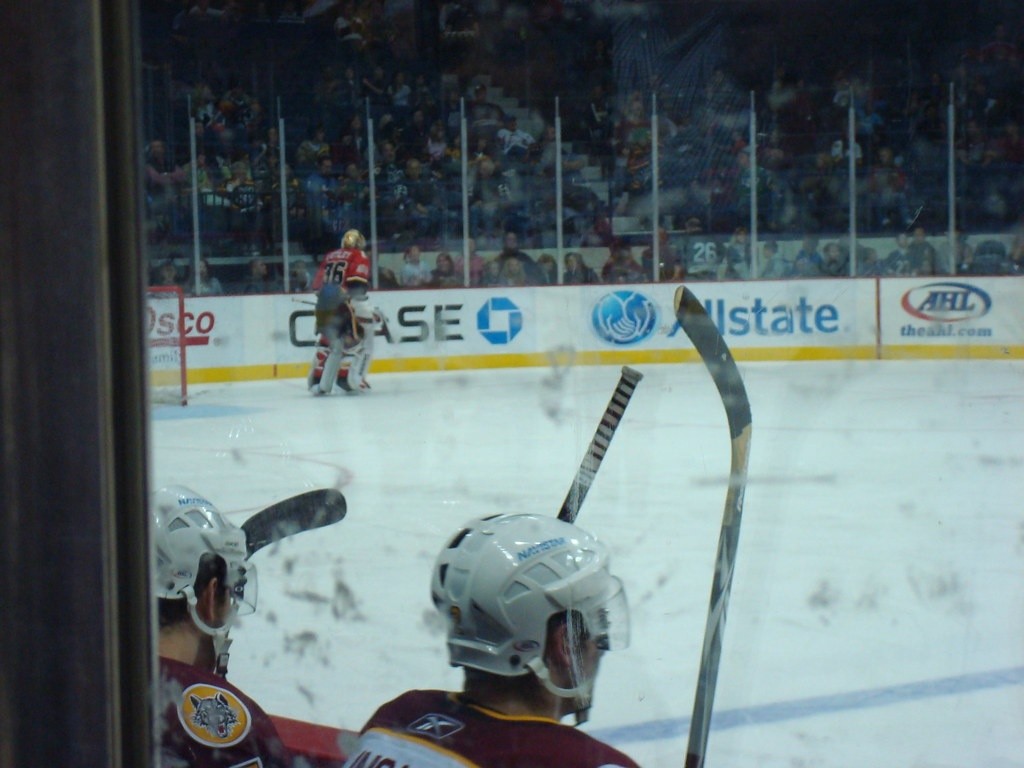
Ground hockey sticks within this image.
[672,282,753,768]
[290,295,318,306]
[552,362,644,526]
[243,487,348,562]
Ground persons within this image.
[151,485,296,768]
[343,513,645,768]
[309,229,371,393]
[137,1,1024,292]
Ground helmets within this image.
[341,229,366,250]
[431,511,620,676]
[151,486,248,599]
